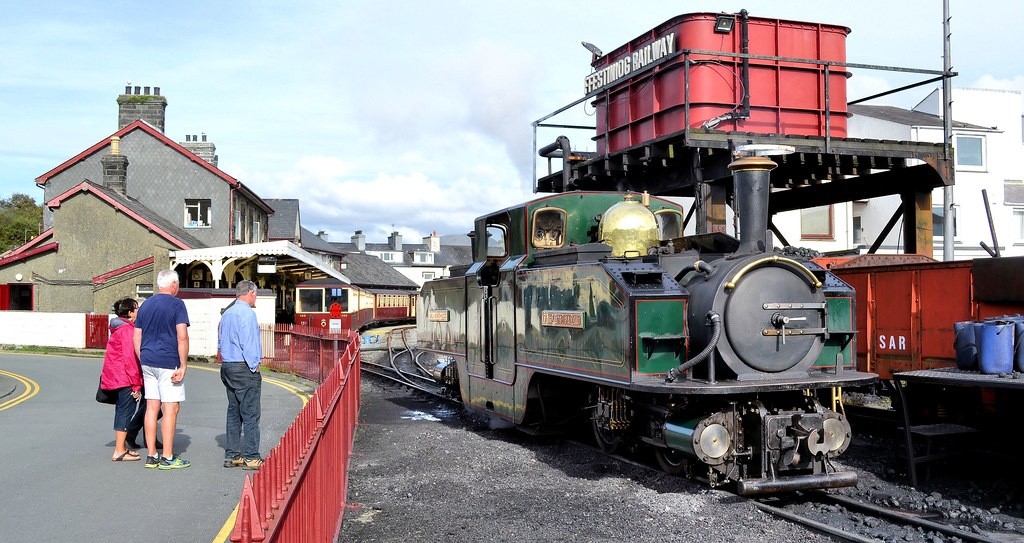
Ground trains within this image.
[295,278,421,331]
[416,158,877,495]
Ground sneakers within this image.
[144,455,160,468]
[223,456,244,467]
[242,458,263,470]
[158,453,190,470]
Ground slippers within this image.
[112,449,141,461]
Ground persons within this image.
[133,267,190,469]
[217,279,266,470]
[100,297,164,462]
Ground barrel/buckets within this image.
[953,314,1024,375]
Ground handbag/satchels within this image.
[96,375,119,404]
